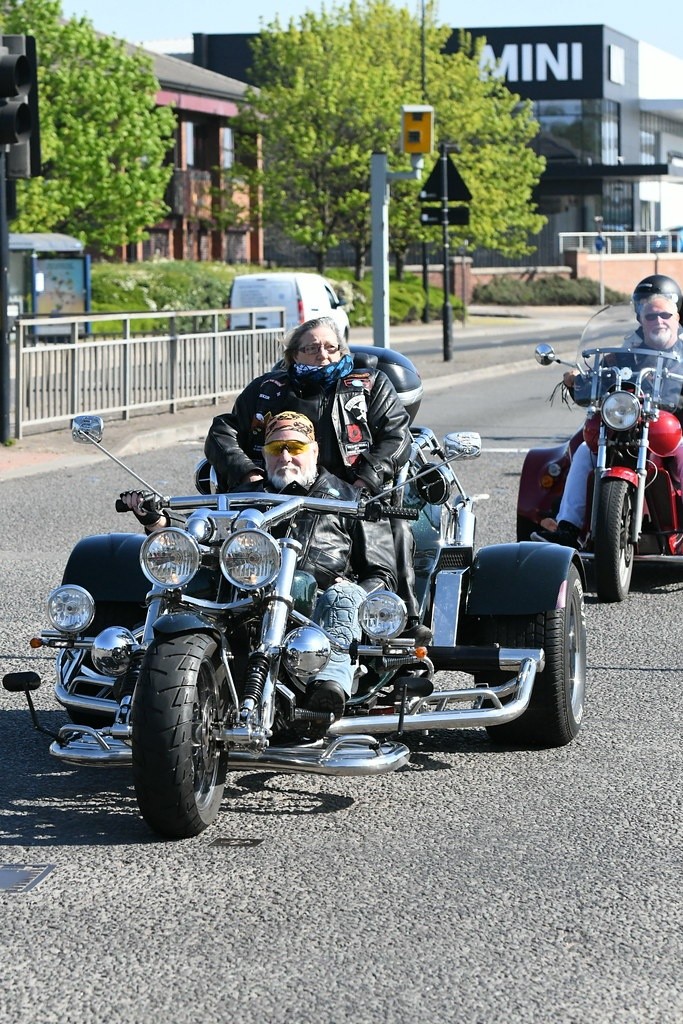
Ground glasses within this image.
[295,344,340,354]
[263,441,310,455]
[643,312,673,320]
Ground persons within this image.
[603,274,682,348]
[530,294,683,559]
[119,412,396,741]
[204,316,433,649]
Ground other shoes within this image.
[308,681,346,738]
[396,625,433,647]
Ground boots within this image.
[530,520,586,552]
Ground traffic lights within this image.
[0,45,31,148]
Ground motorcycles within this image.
[514,302,682,605]
[0,337,590,842]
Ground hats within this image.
[263,410,315,444]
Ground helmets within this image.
[646,410,681,457]
[631,275,682,314]
[583,411,614,455]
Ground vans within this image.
[227,271,351,362]
